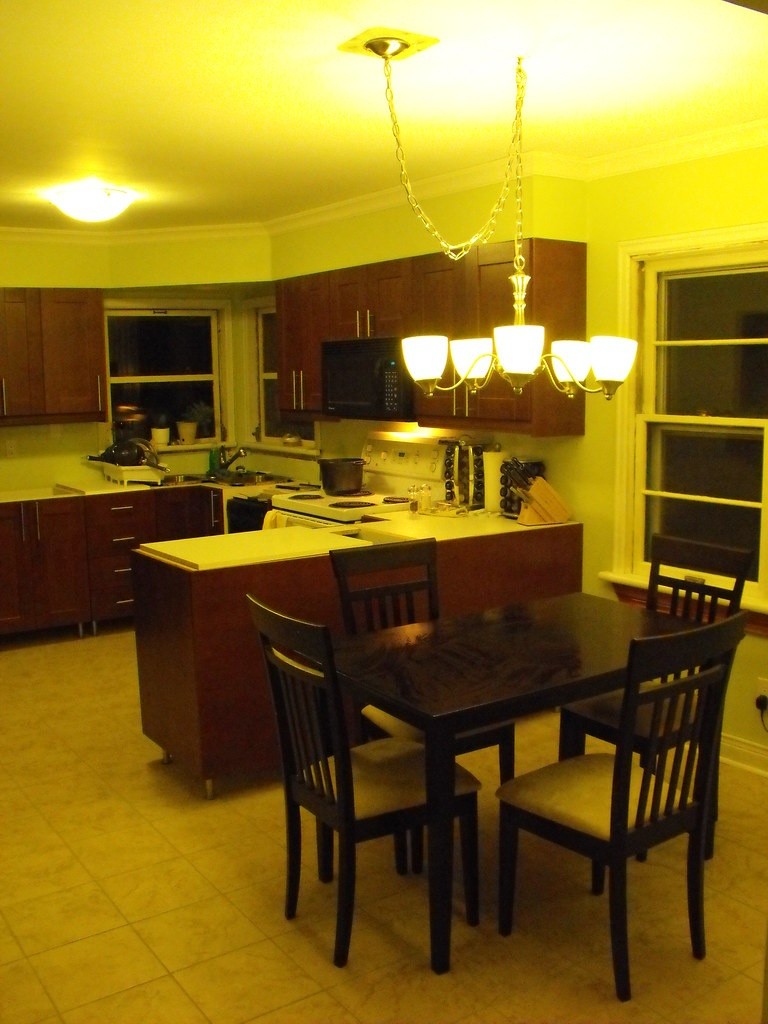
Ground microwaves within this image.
[320,337,416,422]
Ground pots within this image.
[85,438,170,473]
[317,457,366,496]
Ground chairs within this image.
[496,611,749,1001]
[560,536,751,858]
[244,537,517,968]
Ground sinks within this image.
[162,475,201,483]
[219,472,292,485]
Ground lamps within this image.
[337,26,638,402]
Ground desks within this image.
[295,593,708,976]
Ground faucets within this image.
[219,445,248,474]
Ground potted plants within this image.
[150,413,170,446]
[176,402,213,444]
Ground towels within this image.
[262,510,289,530]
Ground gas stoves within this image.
[271,428,457,528]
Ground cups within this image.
[176,421,197,445]
[151,427,170,447]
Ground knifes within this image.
[501,456,536,503]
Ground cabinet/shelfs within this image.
[412,237,586,438]
[0,286,109,427]
[156,486,224,542]
[331,257,412,341]
[275,271,339,422]
[84,489,156,637]
[129,524,584,801]
[0,496,92,639]
